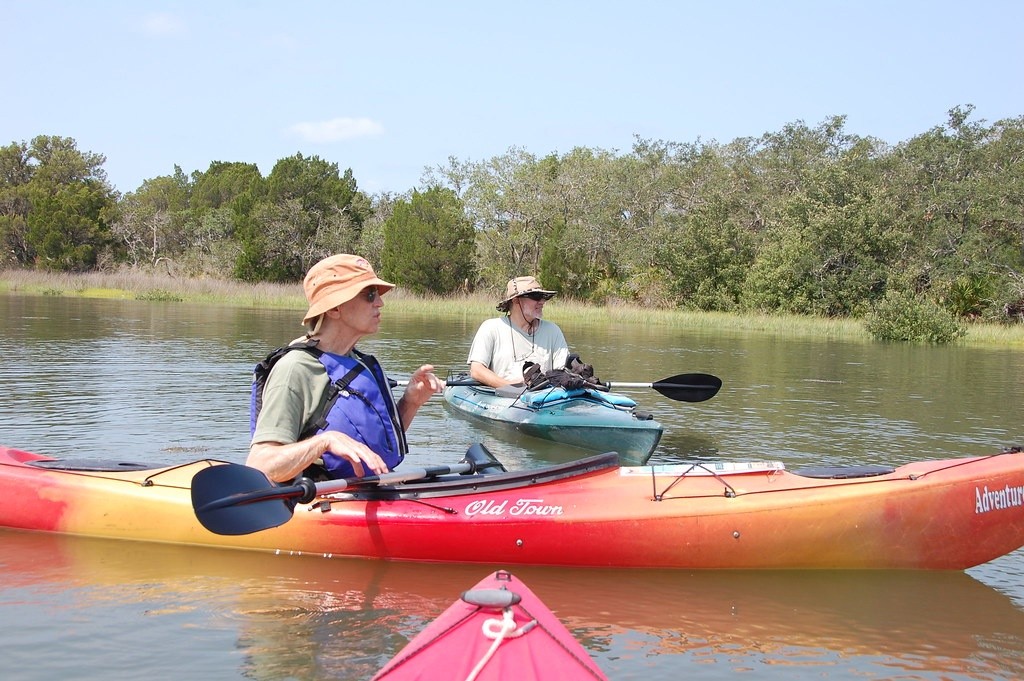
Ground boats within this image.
[369,570,608,681]
[440,373,665,466]
[0,438,1024,574]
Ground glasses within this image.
[518,292,553,302]
[360,286,380,303]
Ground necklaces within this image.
[507,315,535,362]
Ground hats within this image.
[495,275,558,313]
[300,254,397,327]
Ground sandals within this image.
[563,353,612,392]
[522,361,551,392]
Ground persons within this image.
[245,253,446,483]
[465,276,570,392]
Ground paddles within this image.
[189,442,509,538]
[386,372,724,403]
